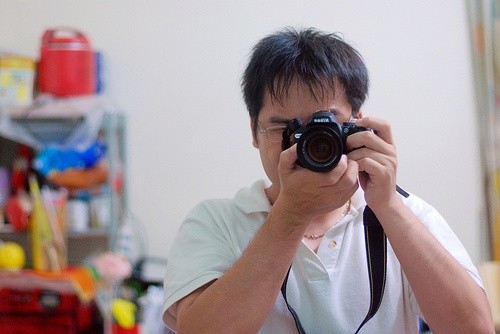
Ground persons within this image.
[161,27,496,334]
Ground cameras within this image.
[282,110,374,173]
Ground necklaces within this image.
[267,183,351,240]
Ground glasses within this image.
[257,120,288,142]
